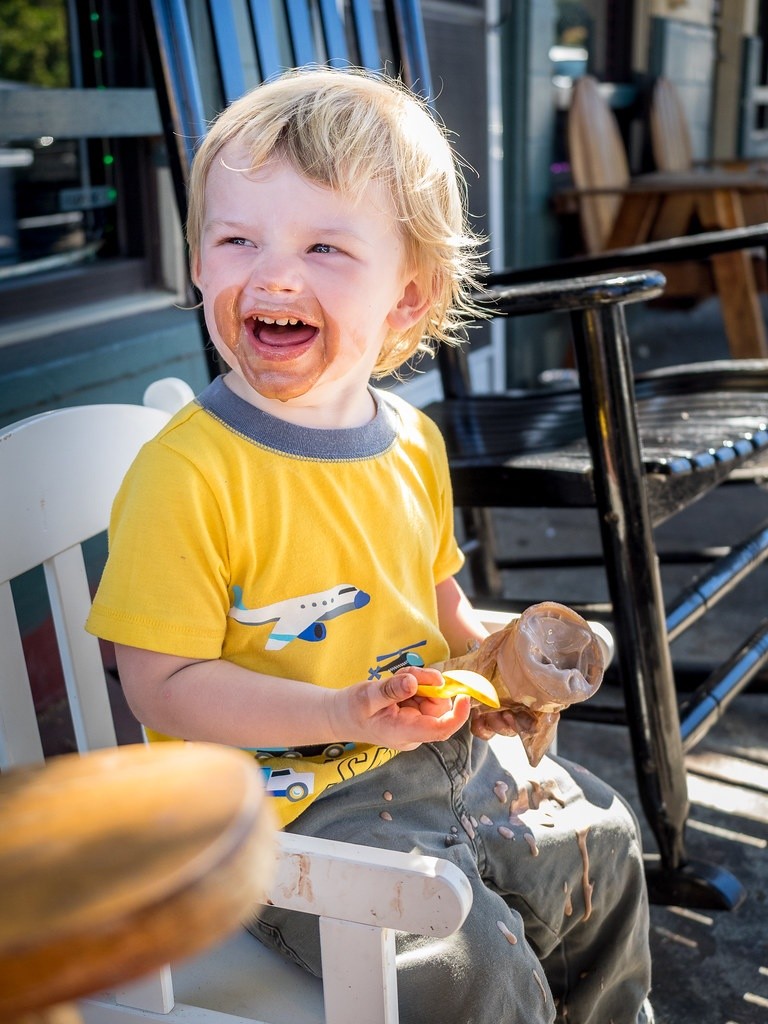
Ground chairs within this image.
[0,378,614,1023]
[140,1,768,908]
[647,73,767,315]
[569,71,768,362]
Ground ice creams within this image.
[424,602,602,766]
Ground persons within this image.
[82,57,659,1024]
